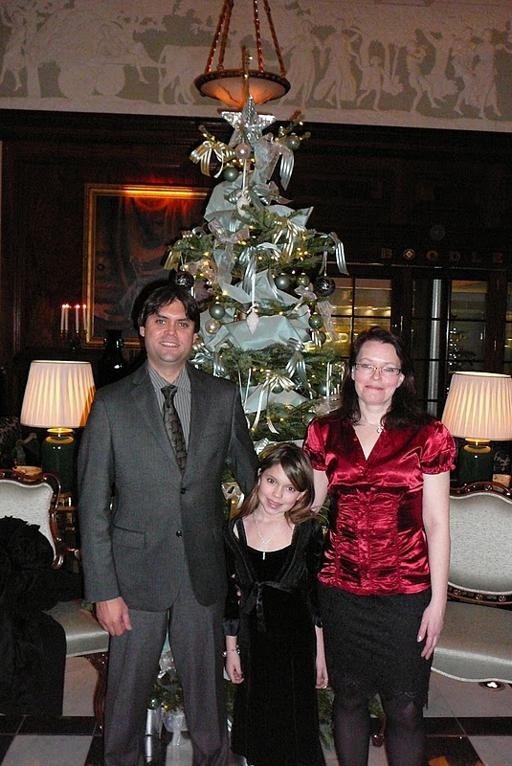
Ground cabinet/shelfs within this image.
[303,251,511,421]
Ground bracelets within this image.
[226,648,237,652]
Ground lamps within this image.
[18,359,96,491]
[436,369,512,494]
[192,1,294,112]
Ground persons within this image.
[226,439,331,763]
[298,328,458,765]
[73,277,263,764]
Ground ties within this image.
[161,386,186,478]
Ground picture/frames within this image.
[74,180,224,355]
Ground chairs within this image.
[368,492,512,749]
[0,468,112,734]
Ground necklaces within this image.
[362,413,384,434]
[250,509,284,561]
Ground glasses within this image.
[353,364,401,375]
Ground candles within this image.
[59,299,89,334]
